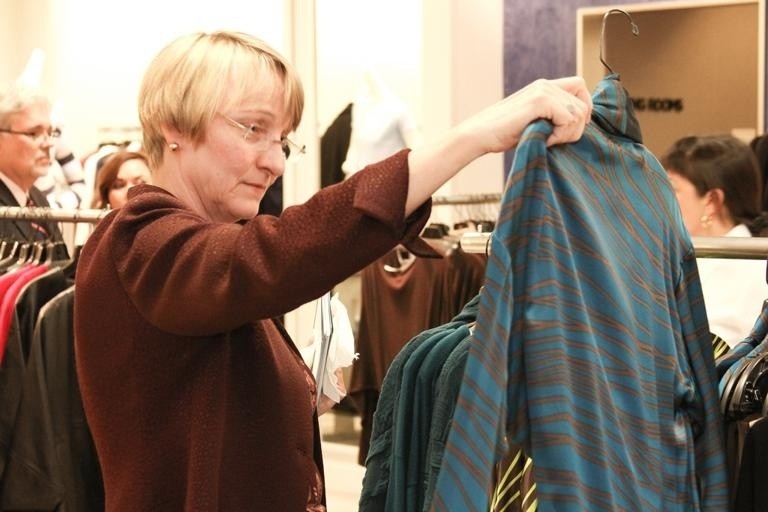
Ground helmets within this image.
[215,109,307,162]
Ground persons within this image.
[659,134,768,351]
[0,87,157,261]
[72,30,591,512]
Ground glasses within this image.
[0,127,61,142]
